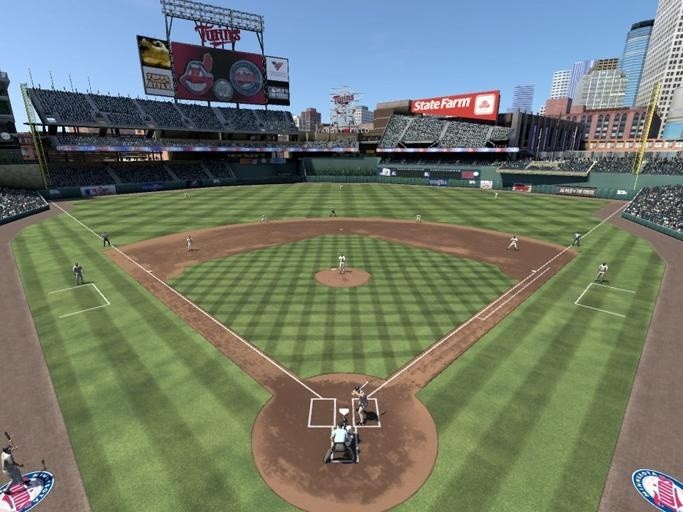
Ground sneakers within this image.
[24,480,30,484]
[4,491,11,495]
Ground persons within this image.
[622,184,683,239]
[0,183,46,223]
[351,385,368,425]
[73,262,84,284]
[308,135,377,176]
[571,232,582,247]
[337,252,346,273]
[333,416,355,450]
[515,148,683,175]
[506,234,520,250]
[25,82,305,189]
[261,214,266,221]
[595,263,609,280]
[101,231,111,247]
[323,420,357,463]
[416,213,422,223]
[330,208,336,217]
[1,445,31,494]
[495,191,499,199]
[378,110,515,176]
[187,234,193,252]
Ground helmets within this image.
[355,386,360,390]
[3,445,11,453]
[343,417,347,423]
